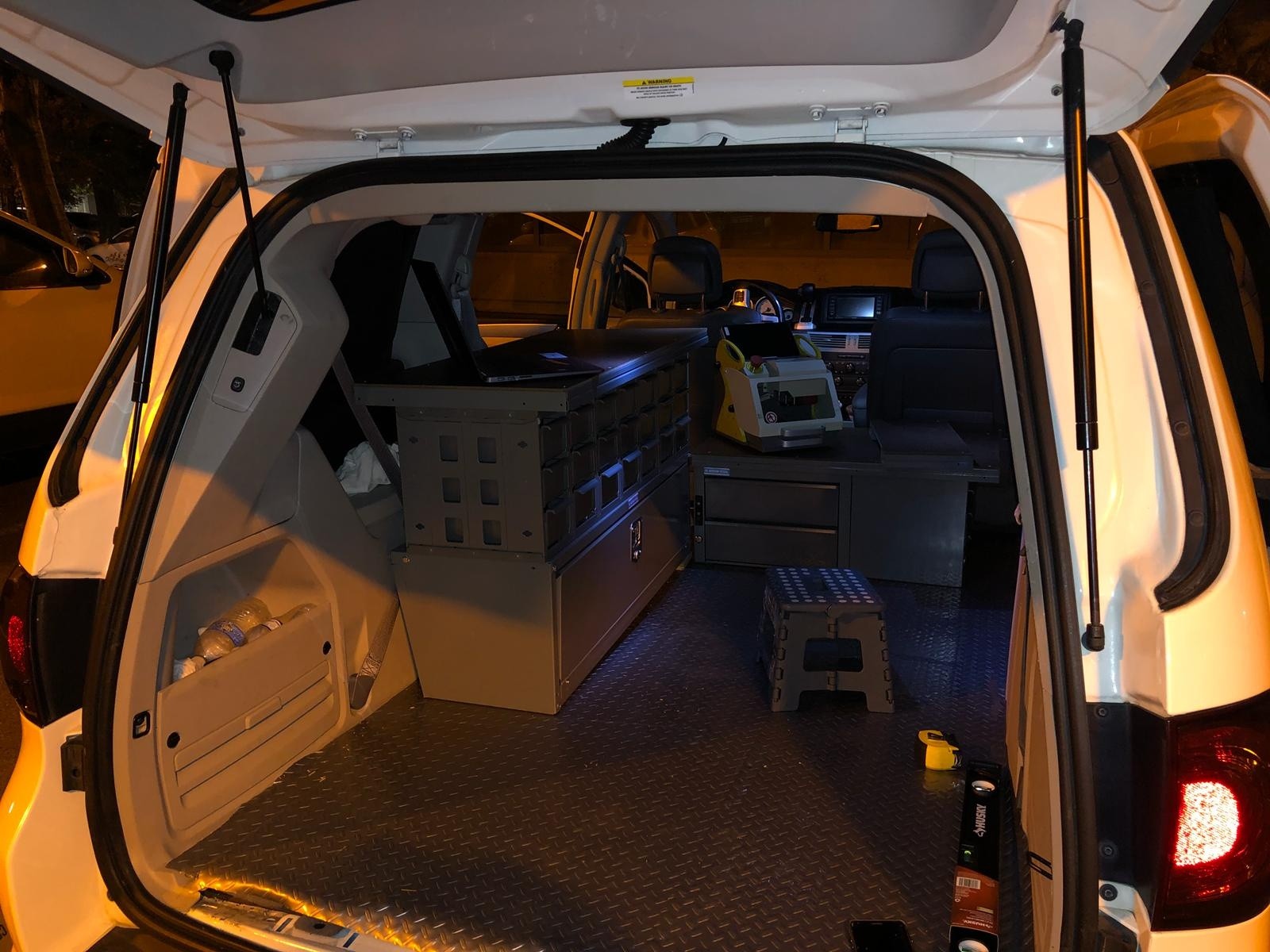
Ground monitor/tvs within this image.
[827,295,887,321]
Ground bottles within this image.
[190,597,271,662]
[232,603,317,651]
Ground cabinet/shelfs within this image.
[351,328,710,714]
[693,421,998,588]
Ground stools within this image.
[757,566,895,713]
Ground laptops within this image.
[410,261,605,386]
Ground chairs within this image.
[616,235,760,341]
[869,230,1007,418]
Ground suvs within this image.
[0,0,1269,950]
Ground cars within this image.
[622,211,721,275]
[0,207,125,486]
[85,226,135,272]
[68,220,100,251]
